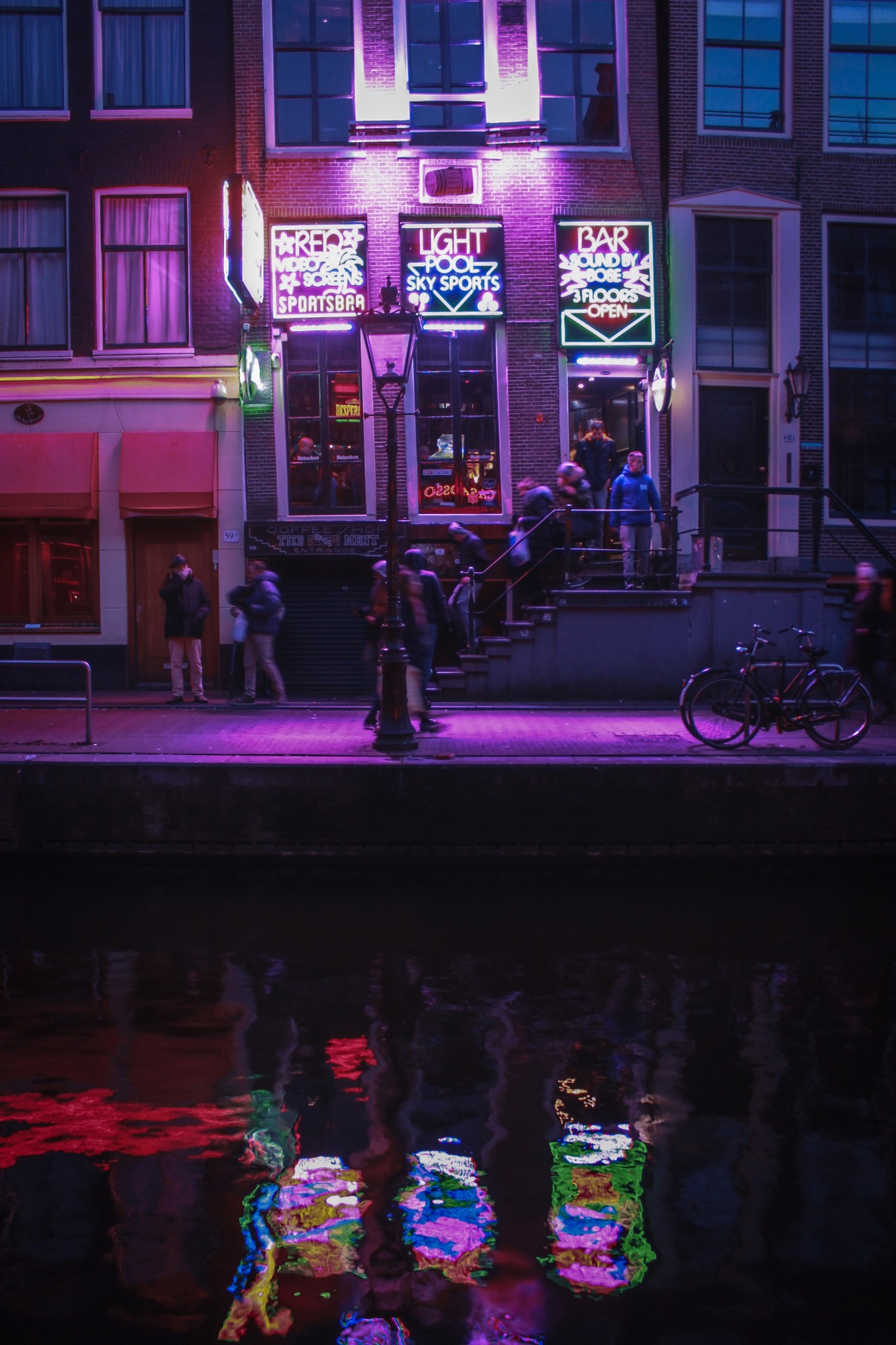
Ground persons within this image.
[609,451,665,590]
[227,561,286,704]
[420,445,430,460]
[837,562,887,723]
[289,437,343,516]
[573,420,620,552]
[365,549,446,731]
[512,477,556,607]
[556,461,599,590]
[230,579,285,703]
[448,521,488,654]
[159,554,211,704]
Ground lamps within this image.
[783,355,813,423]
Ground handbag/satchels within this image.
[232,610,248,643]
[509,522,530,566]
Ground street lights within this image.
[349,273,423,752]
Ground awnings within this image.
[0,432,99,519]
[119,431,219,519]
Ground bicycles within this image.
[677,623,876,751]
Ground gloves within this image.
[179,569,189,580]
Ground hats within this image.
[169,554,186,568]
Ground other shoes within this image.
[194,695,209,703]
[363,718,377,729]
[233,694,255,704]
[625,580,634,589]
[165,696,183,704]
[419,719,441,731]
[271,695,288,704]
[635,579,644,588]
